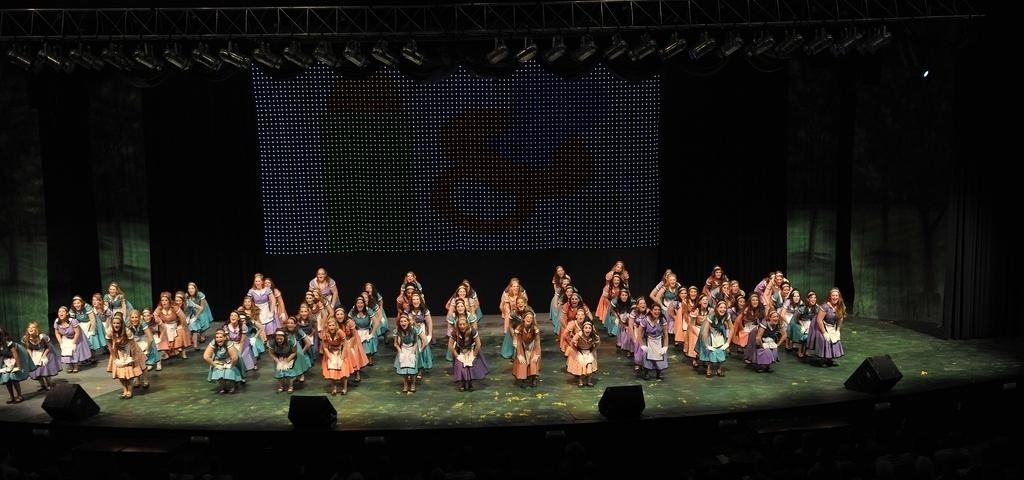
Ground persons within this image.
[2,260,847,405]
[331,418,1008,480]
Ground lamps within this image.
[598,385,646,424]
[39,379,101,425]
[285,391,340,430]
[844,350,904,402]
[4,0,898,79]
